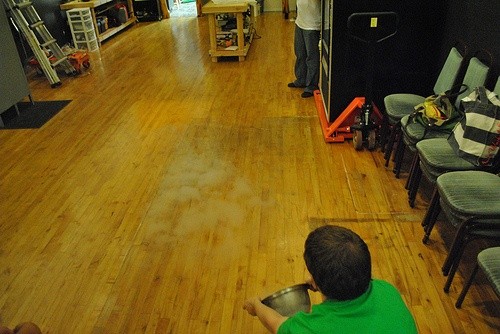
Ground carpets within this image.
[0,100,73,130]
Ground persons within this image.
[288,0,323,98]
[242,225,418,334]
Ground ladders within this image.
[1,0,77,89]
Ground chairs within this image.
[384,47,500,309]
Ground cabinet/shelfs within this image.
[66,7,99,52]
[201,0,257,62]
[60,0,137,48]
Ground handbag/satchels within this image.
[402,81,469,132]
[446,84,500,166]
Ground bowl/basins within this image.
[260,283,318,317]
